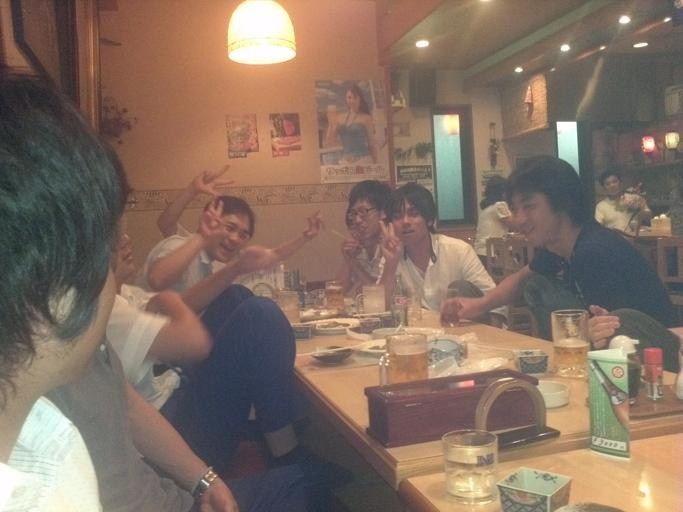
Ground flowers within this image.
[612,181,648,211]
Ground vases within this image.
[629,213,638,230]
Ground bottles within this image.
[606,334,639,407]
[641,344,664,400]
[588,359,632,436]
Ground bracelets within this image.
[193,468,219,498]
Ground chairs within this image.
[485,233,541,339]
[654,236,681,307]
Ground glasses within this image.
[348,207,379,220]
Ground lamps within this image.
[225,0,298,69]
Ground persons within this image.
[0,71,128,512]
[96,209,356,490]
[47,336,238,511]
[323,81,379,165]
[594,172,652,233]
[132,162,328,436]
[328,175,519,328]
[440,157,679,374]
[273,118,300,156]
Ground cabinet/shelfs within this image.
[500,63,655,141]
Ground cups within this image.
[440,428,498,506]
[548,308,591,378]
[269,261,469,369]
[378,332,429,385]
[652,217,671,237]
[325,104,335,126]
